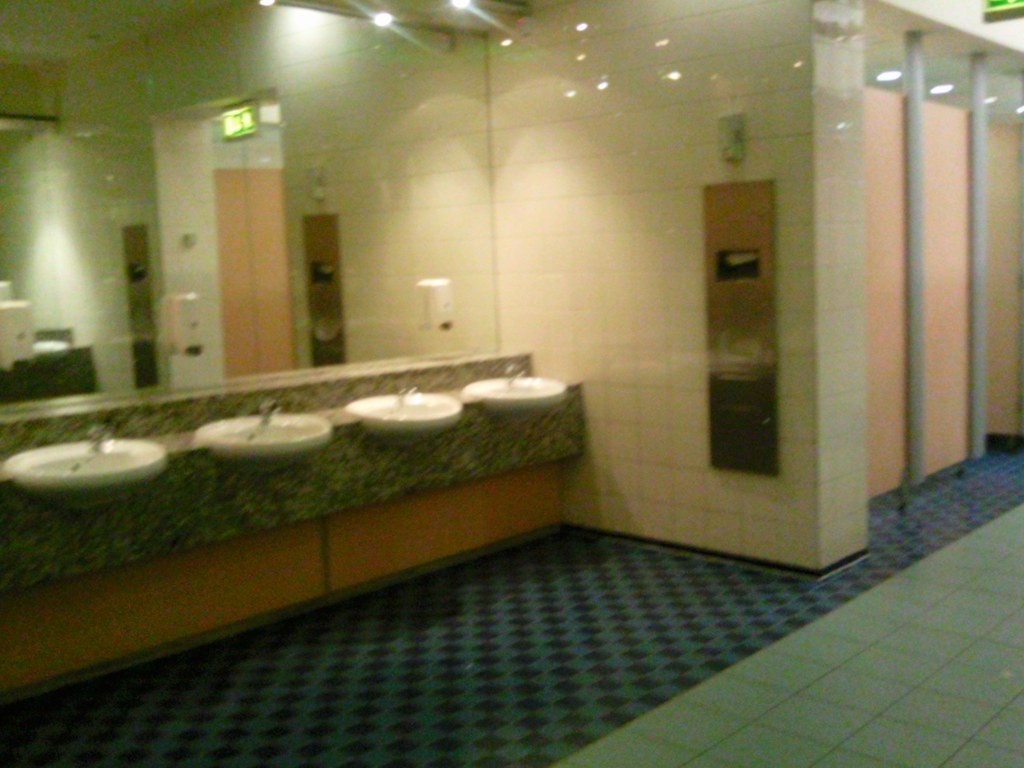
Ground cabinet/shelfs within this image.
[1,456,572,708]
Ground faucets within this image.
[85,421,118,458]
[395,384,420,409]
[259,397,283,429]
[506,368,527,390]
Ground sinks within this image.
[461,377,569,410]
[191,414,336,456]
[5,440,168,489]
[347,393,465,435]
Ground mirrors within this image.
[1,0,497,411]
